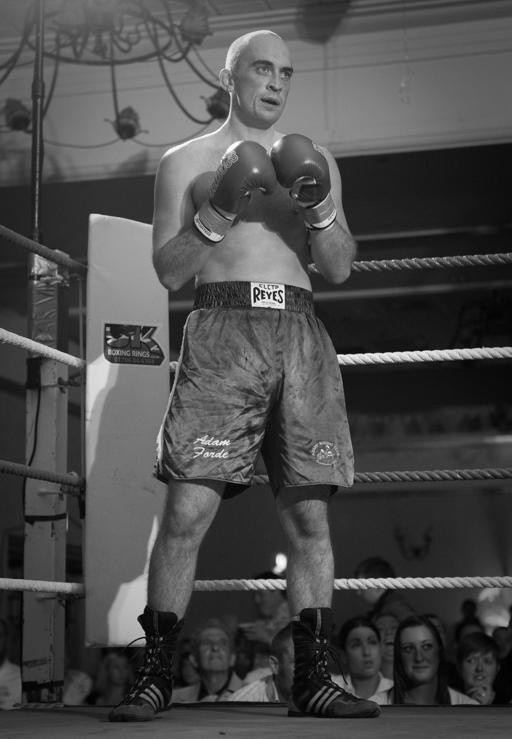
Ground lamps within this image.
[0,0,230,148]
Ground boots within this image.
[108,605,186,722]
[287,606,383,719]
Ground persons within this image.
[145,554,511,705]
[108,28,382,719]
[97,652,136,705]
